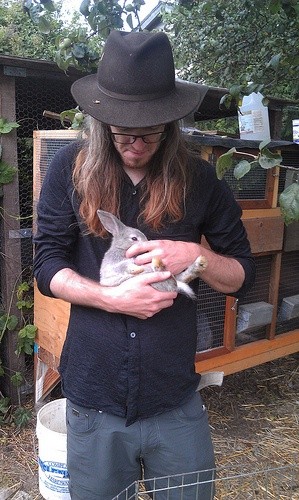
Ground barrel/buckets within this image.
[32,396,74,500]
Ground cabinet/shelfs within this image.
[35,129,299,407]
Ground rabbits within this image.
[97,209,208,300]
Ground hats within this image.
[70,30,209,129]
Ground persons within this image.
[33,30,255,500]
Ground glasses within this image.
[105,125,170,147]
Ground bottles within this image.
[238,81,272,143]
